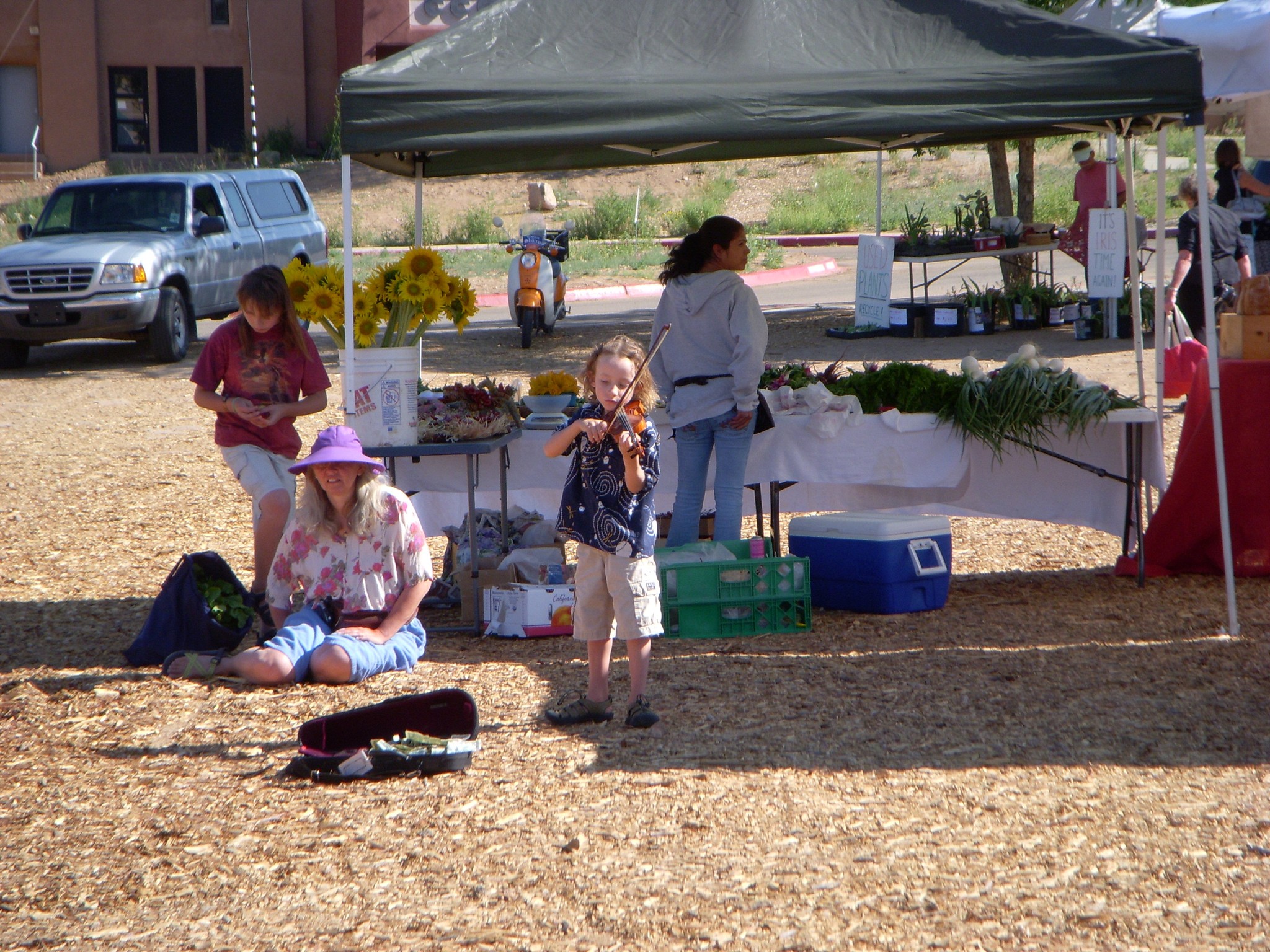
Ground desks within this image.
[874,244,1080,305]
[1115,358,1270,587]
[359,368,1161,584]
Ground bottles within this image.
[749,532,765,558]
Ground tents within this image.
[1156,0,1270,503]
[337,0,1242,637]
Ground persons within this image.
[162,188,207,229]
[190,263,332,632]
[162,424,434,688]
[1066,141,1126,322]
[1164,172,1252,344]
[1232,159,1270,275]
[1208,139,1257,278]
[543,335,665,728]
[647,214,769,547]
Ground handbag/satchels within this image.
[128,550,255,658]
[757,381,865,438]
[1227,168,1267,219]
[439,503,572,584]
[420,577,461,608]
[1163,305,1209,399]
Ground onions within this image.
[961,344,1109,392]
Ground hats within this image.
[288,426,388,474]
[1072,145,1093,162]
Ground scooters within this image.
[492,210,575,349]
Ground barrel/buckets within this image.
[925,303,965,337]
[888,303,924,338]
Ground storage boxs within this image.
[440,527,573,639]
[1219,312,1270,358]
[789,512,956,619]
[645,536,816,638]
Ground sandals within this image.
[547,695,614,722]
[624,694,659,726]
[164,649,231,680]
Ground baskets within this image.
[658,556,813,638]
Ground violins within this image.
[605,398,650,460]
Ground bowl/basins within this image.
[522,394,571,413]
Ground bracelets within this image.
[232,397,241,414]
[222,394,229,413]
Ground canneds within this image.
[750,535,764,558]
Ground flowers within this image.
[280,244,482,347]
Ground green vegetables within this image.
[193,563,256,630]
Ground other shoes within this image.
[257,628,276,647]
[249,588,275,625]
[1173,401,1187,412]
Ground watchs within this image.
[1166,284,1180,292]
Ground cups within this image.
[1058,227,1066,241]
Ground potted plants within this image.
[825,278,1165,340]
[892,191,1025,253]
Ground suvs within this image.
[0,168,330,371]
